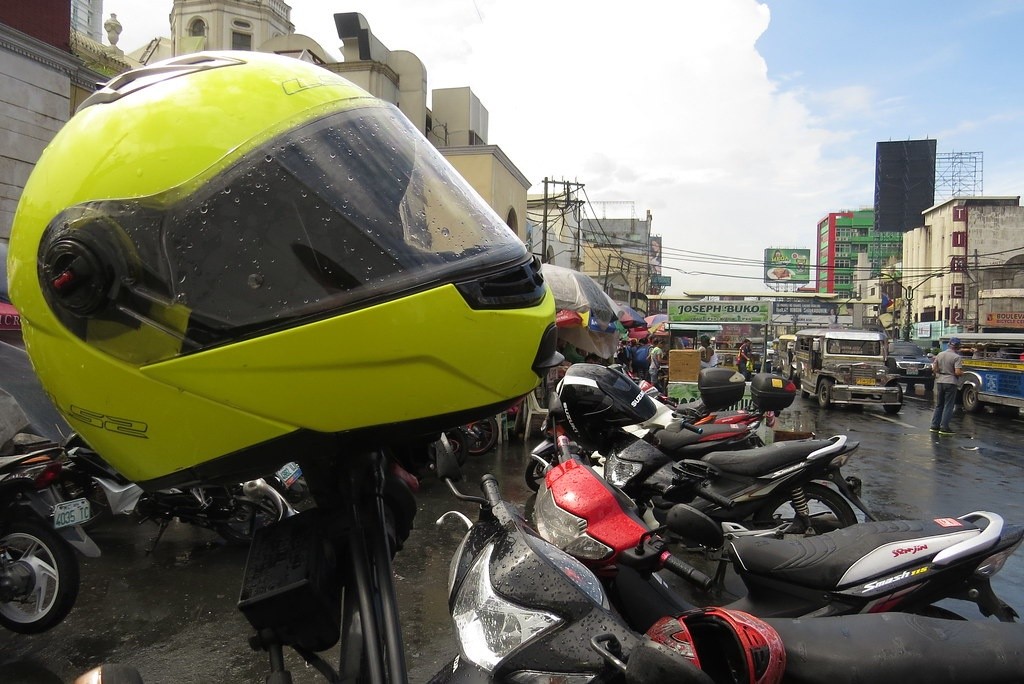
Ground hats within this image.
[950,337,960,347]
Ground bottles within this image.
[746,358,753,371]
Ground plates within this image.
[767,268,795,281]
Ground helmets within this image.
[7,50,565,491]
[644,606,786,684]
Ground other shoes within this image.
[939,427,956,435]
[929,428,940,433]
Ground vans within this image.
[794,329,904,415]
[938,334,1024,416]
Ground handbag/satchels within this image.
[746,360,754,372]
[659,369,665,375]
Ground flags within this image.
[881,291,893,311]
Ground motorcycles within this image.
[0,447,101,637]
[58,409,500,559]
[421,363,1024,684]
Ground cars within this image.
[887,342,936,391]
[744,334,799,380]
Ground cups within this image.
[796,259,806,272]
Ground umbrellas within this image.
[541,263,668,335]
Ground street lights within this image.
[814,294,862,324]
[877,273,948,344]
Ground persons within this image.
[616,335,714,386]
[932,337,964,435]
[737,338,752,381]
